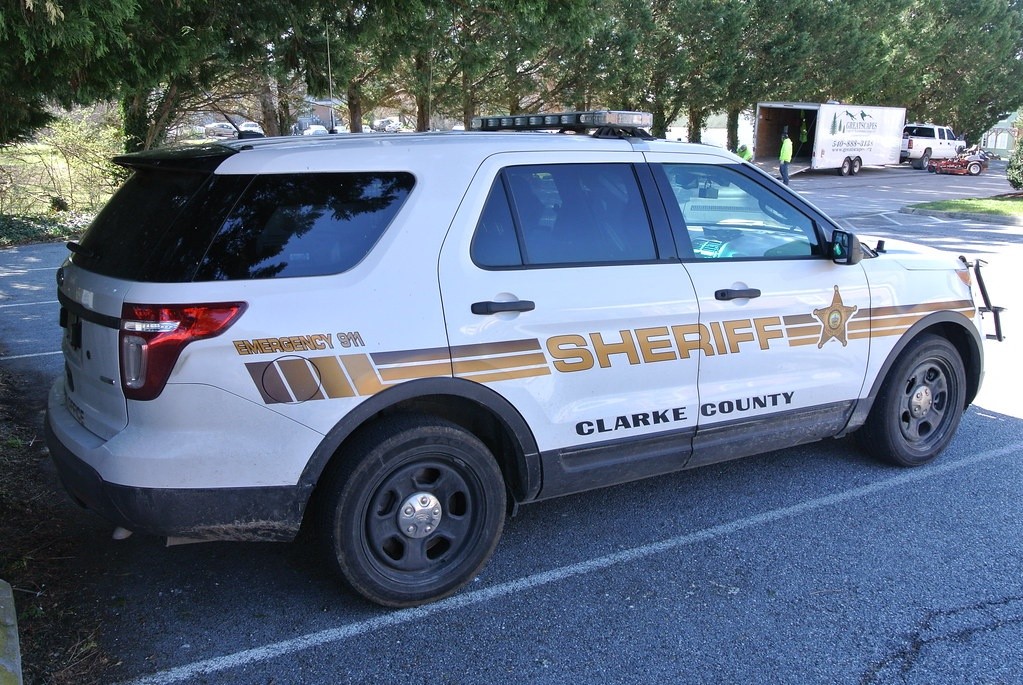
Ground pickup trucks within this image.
[899,122,967,170]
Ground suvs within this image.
[40,109,1007,610]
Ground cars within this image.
[984,151,1001,161]
[165,111,416,138]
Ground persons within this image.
[735,145,752,162]
[779,134,792,185]
